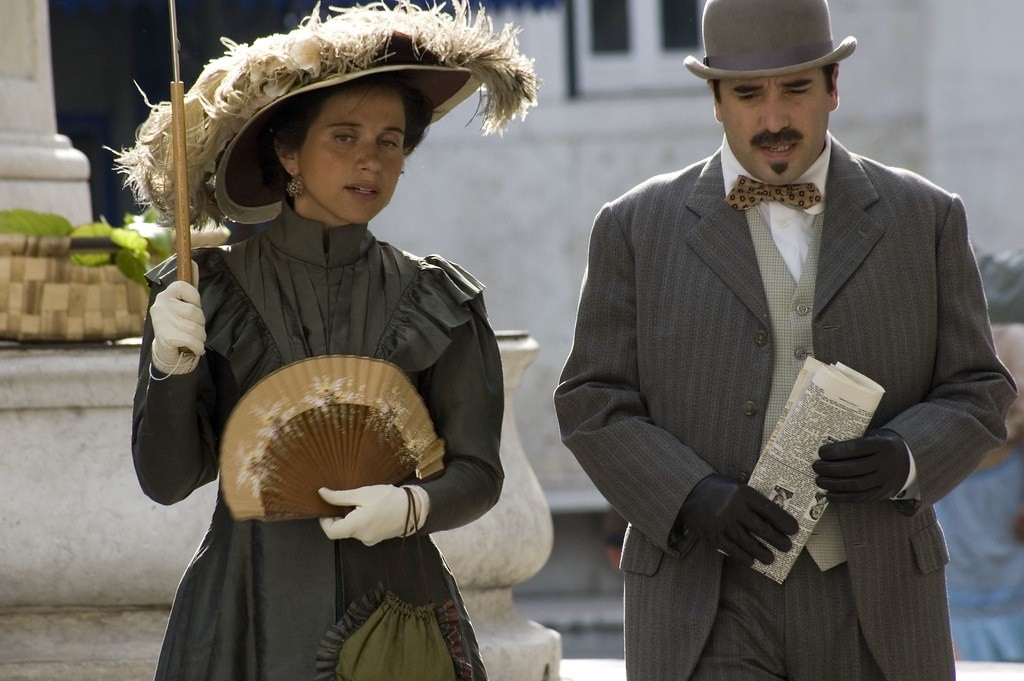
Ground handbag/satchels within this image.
[315,488,473,680]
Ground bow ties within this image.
[725,175,824,211]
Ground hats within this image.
[684,0,857,78]
[123,0,540,225]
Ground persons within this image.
[553,0,1018,680]
[115,1,537,680]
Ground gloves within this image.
[316,483,428,547]
[150,260,207,376]
[812,427,909,504]
[678,473,800,567]
